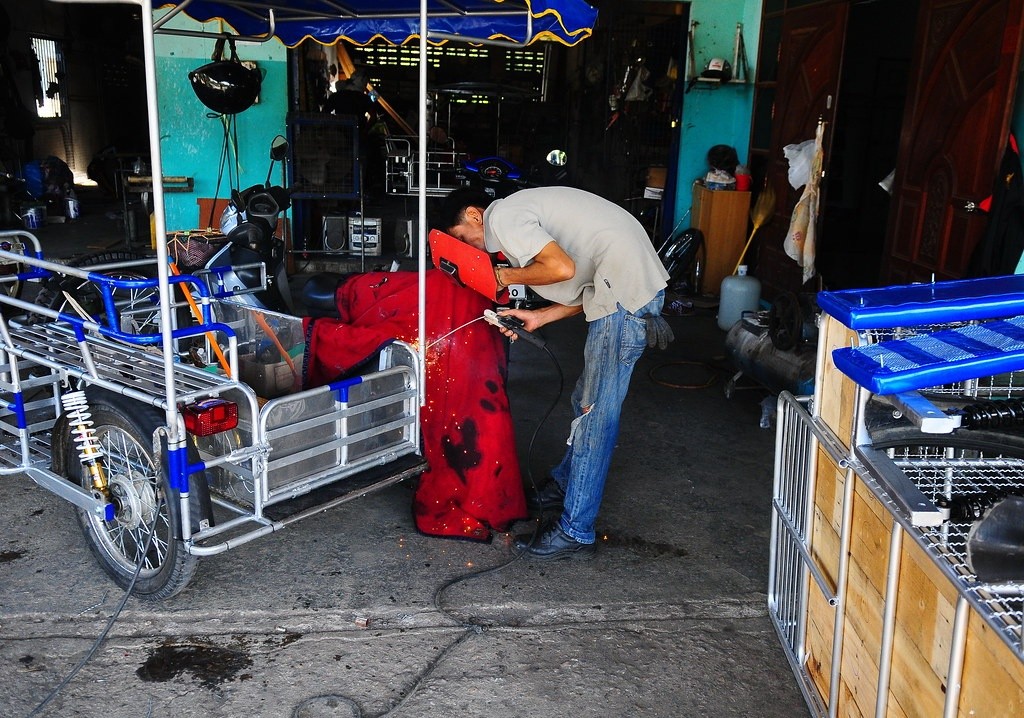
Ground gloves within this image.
[642,313,675,349]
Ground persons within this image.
[441,187,671,561]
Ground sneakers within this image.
[511,519,598,562]
[526,476,565,510]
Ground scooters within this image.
[176,135,363,352]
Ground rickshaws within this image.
[1,0,539,607]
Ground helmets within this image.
[188,60,262,114]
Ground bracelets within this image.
[496,267,507,287]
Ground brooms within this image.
[252,311,302,393]
[732,190,775,275]
[167,256,268,412]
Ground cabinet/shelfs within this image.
[690,184,751,297]
